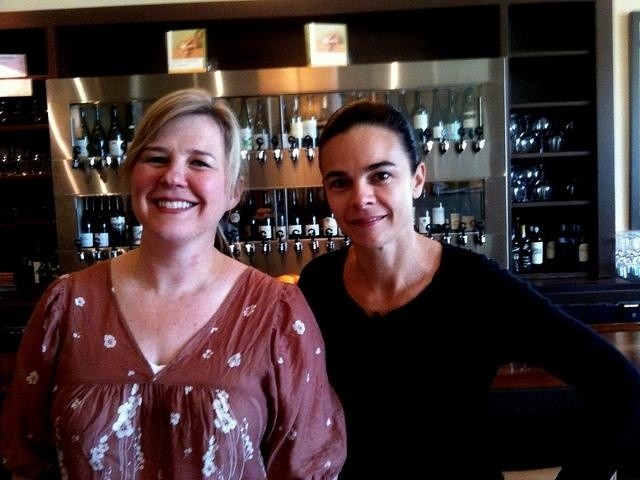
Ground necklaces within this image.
[371,309,382,317]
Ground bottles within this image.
[76,184,487,250]
[506,102,595,275]
[66,85,487,166]
[0,87,52,177]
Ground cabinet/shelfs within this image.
[0,0,617,301]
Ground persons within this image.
[6,91,350,480]
[296,94,639,480]
